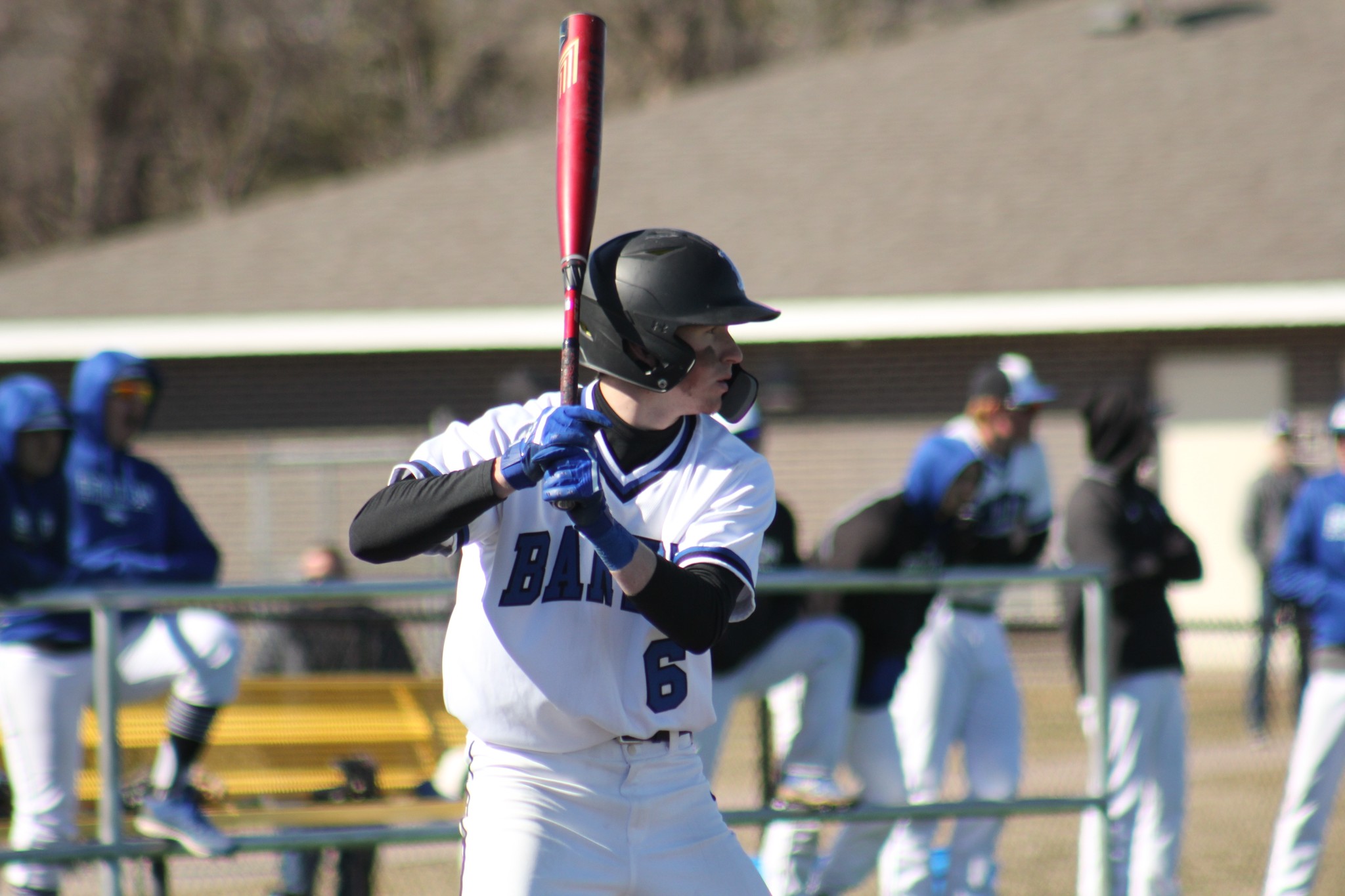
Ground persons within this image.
[0,352,240,895]
[350,225,783,888]
[877,352,1059,896]
[240,541,419,895]
[1052,384,1205,895]
[1238,396,1345,896]
[687,351,864,816]
[756,438,982,896]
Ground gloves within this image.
[531,445,614,538]
[500,406,614,490]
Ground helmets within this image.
[577,228,782,424]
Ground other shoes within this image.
[769,772,861,814]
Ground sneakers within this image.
[133,786,237,858]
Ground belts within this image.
[615,730,693,745]
[952,601,995,614]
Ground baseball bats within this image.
[557,9,604,511]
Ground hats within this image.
[107,368,153,397]
[1267,413,1299,443]
[15,414,72,433]
[709,398,764,446]
[973,355,1058,407]
[293,551,345,581]
[1082,387,1154,463]
[1327,397,1345,434]
